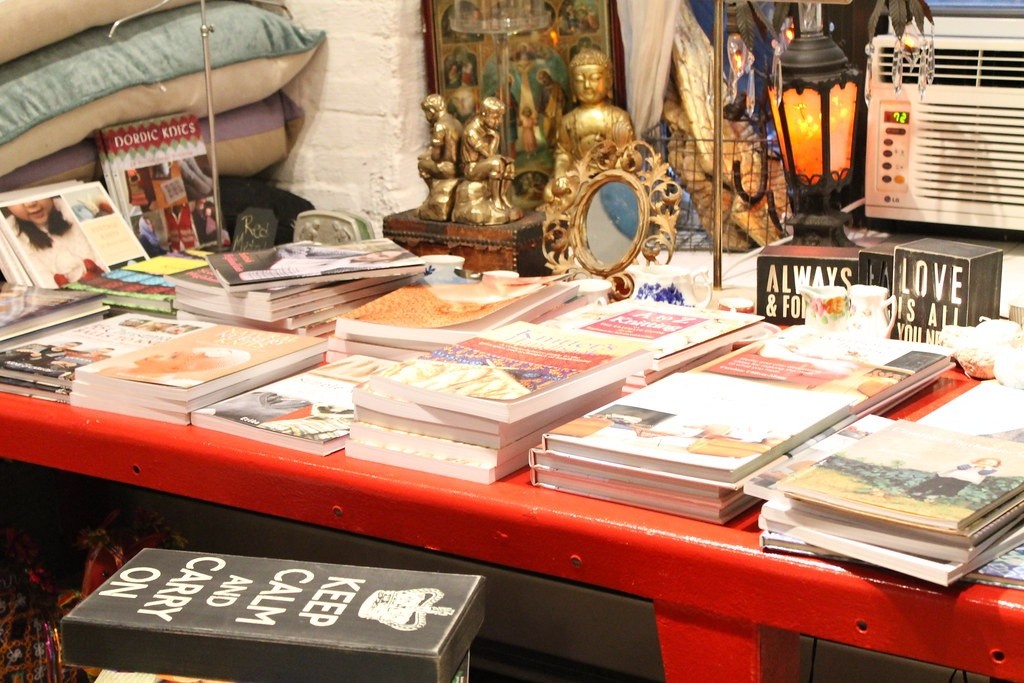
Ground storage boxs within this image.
[384,204,555,280]
[63,541,489,683]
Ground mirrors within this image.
[542,138,684,304]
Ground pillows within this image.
[0,0,327,178]
[0,89,304,191]
[0,0,208,64]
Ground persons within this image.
[36,359,82,371]
[228,245,414,285]
[192,198,216,244]
[544,47,634,203]
[417,94,464,187]
[6,197,105,288]
[100,348,250,385]
[139,215,167,258]
[907,458,999,498]
[2,342,82,366]
[462,97,515,210]
[80,346,113,362]
[203,201,216,236]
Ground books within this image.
[0,114,1024,586]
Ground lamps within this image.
[766,29,863,252]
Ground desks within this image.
[0,369,1024,683]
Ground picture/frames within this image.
[419,0,627,211]
